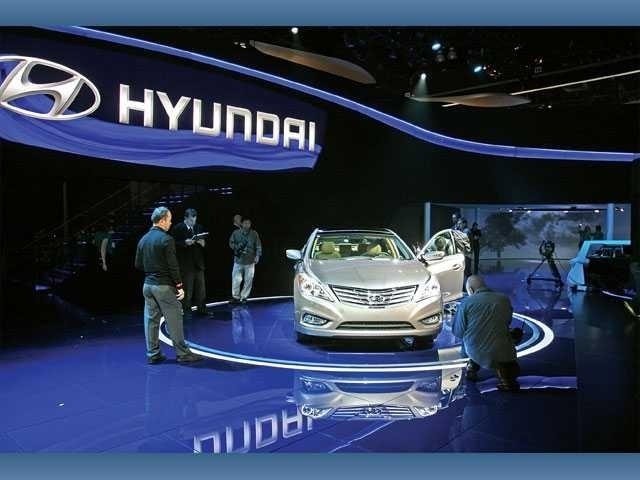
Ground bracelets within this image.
[175,284,185,290]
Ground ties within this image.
[188,227,193,234]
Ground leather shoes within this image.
[229,297,238,303]
[240,298,247,305]
[183,307,195,315]
[149,353,167,365]
[499,381,521,391]
[465,363,478,379]
[177,350,205,362]
[196,307,214,316]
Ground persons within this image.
[427,210,482,276]
[223,213,265,298]
[170,208,215,317]
[623,215,640,318]
[228,305,260,392]
[94,212,121,272]
[590,225,604,240]
[576,223,591,250]
[133,206,205,365]
[228,215,262,305]
[451,274,521,391]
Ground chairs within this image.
[313,241,384,260]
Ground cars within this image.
[293,342,466,424]
[284,223,466,350]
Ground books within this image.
[192,231,210,241]
